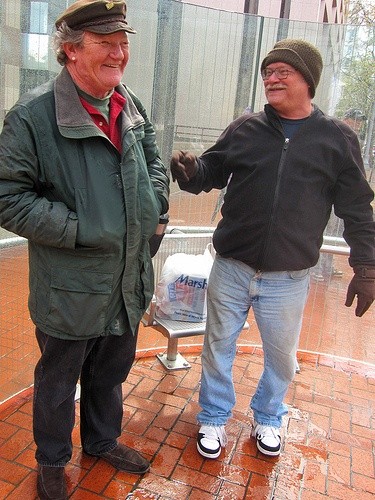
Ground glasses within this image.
[260,67,296,80]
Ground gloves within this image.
[344,264,375,317]
[169,150,198,181]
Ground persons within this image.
[0,0,171,500]
[170,40,375,459]
[307,109,367,281]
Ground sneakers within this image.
[196,423,226,458]
[252,417,281,456]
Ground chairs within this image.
[141,233,250,371]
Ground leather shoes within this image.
[81,444,150,473]
[35,464,67,500]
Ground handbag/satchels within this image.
[154,243,215,322]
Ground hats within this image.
[260,40,323,97]
[55,0,135,35]
[343,108,368,121]
